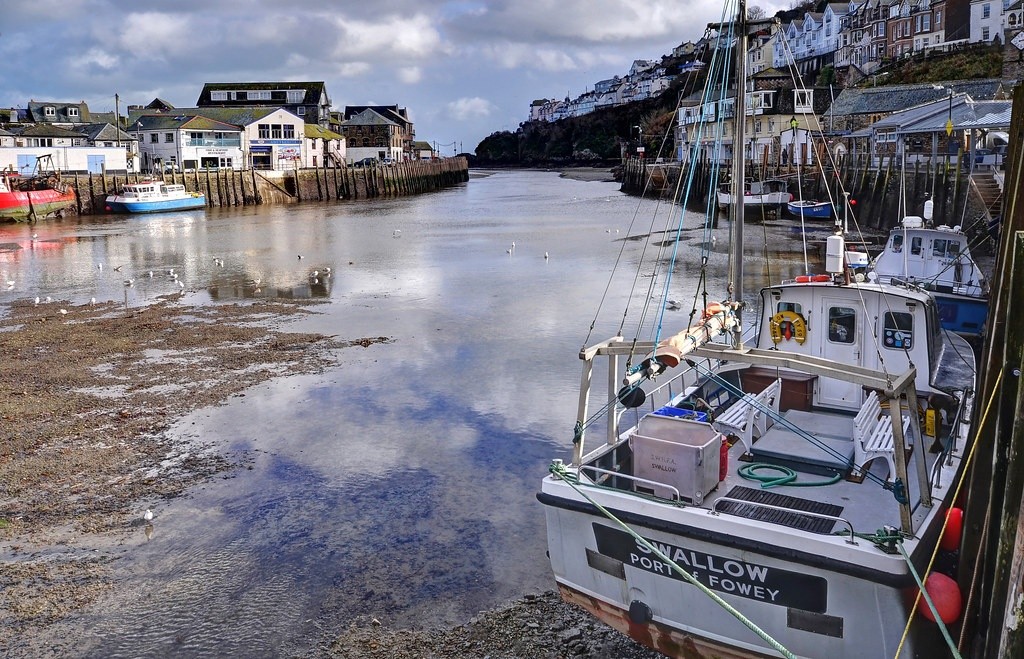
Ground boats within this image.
[105,161,207,214]
[0,163,78,223]
[860,127,991,349]
[716,171,793,219]
[786,199,832,220]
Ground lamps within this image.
[868,272,879,283]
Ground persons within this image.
[790,115,796,128]
[153,161,158,170]
[257,160,263,166]
[782,147,788,165]
[208,159,213,165]
[393,159,396,166]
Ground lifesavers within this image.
[796,273,831,283]
[770,311,806,344]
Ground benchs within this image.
[976,154,1003,172]
[853,390,911,483]
[713,378,782,456]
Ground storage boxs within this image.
[653,406,708,421]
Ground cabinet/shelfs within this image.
[743,367,819,412]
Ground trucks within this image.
[150,162,179,174]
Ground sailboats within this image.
[533,1,1005,659]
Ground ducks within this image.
[512,242,515,247]
[298,255,304,259]
[32,234,38,238]
[253,278,261,284]
[544,252,549,258]
[310,271,319,278]
[393,230,401,236]
[506,249,511,253]
[212,256,223,267]
[7,263,185,315]
[322,268,330,273]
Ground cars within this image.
[420,157,433,162]
[354,157,378,168]
[379,157,396,167]
